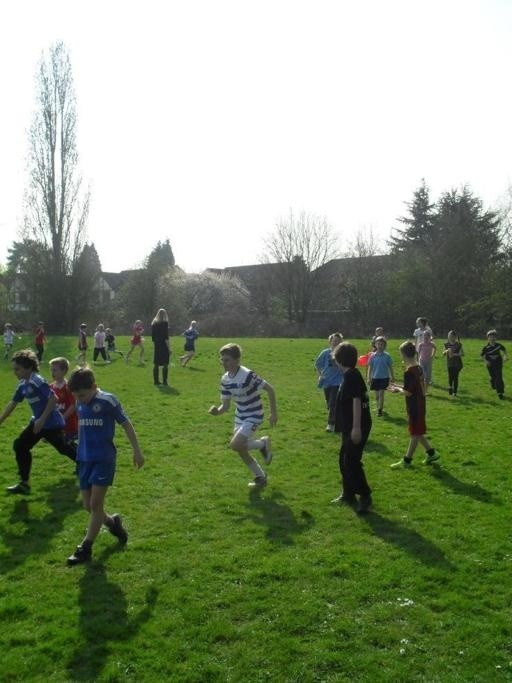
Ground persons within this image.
[481,329,509,399]
[34,321,45,363]
[180,321,199,367]
[92,324,111,364]
[0,349,145,564]
[151,309,171,385]
[316,317,465,512]
[104,327,124,360]
[124,320,146,366]
[3,323,21,358]
[76,323,88,364]
[209,343,278,487]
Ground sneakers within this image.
[330,493,358,506]
[355,497,372,514]
[247,473,268,488]
[108,513,127,544]
[421,449,441,466]
[67,544,92,564]
[259,435,272,465]
[5,482,31,495]
[325,424,335,431]
[389,458,412,469]
